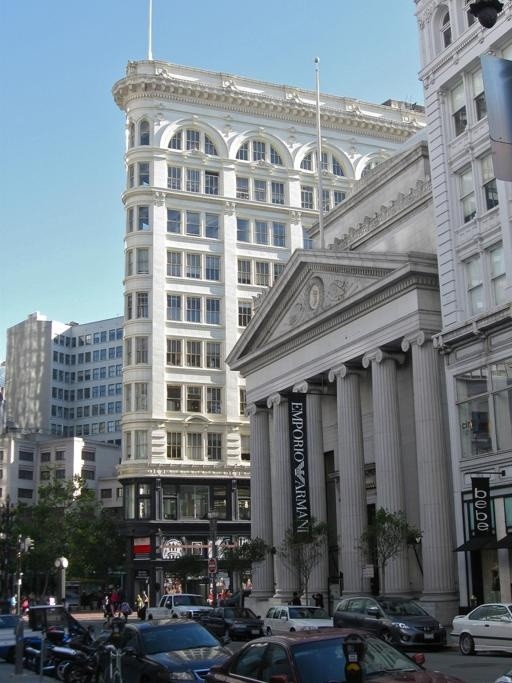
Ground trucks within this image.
[0,605,87,663]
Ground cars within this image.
[197,606,264,640]
[205,627,467,683]
[448,602,512,656]
[105,617,234,683]
[260,604,334,637]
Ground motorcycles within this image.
[19,626,126,683]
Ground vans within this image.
[332,593,448,650]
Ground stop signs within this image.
[207,557,218,575]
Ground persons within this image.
[10,593,17,615]
[20,596,29,615]
[102,589,148,621]
[312,593,324,608]
[293,592,301,606]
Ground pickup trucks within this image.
[145,592,215,621]
